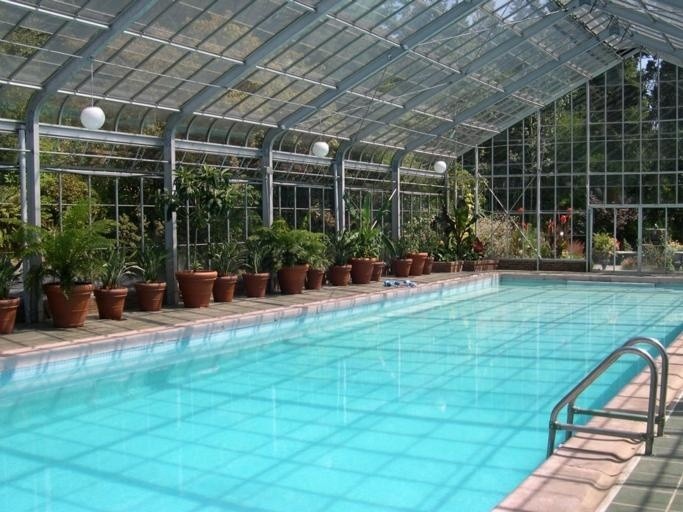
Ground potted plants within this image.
[0,196,497,335]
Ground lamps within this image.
[80,54,105,130]
[433,152,446,174]
[311,106,328,159]
